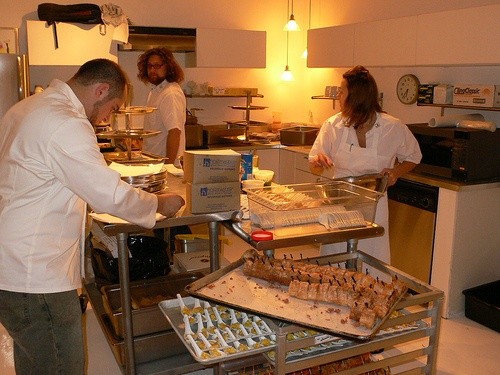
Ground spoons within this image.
[251,205,367,229]
[176,292,277,359]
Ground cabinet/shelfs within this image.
[25,20,267,68]
[307,4,500,68]
[78,94,445,375]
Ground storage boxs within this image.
[279,126,320,145]
[173,240,223,254]
[185,182,243,214]
[201,125,246,144]
[433,84,452,104]
[185,124,203,148]
[418,83,435,103]
[462,279,500,334]
[184,148,241,184]
[173,252,231,273]
[453,83,500,108]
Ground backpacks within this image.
[99,3,128,36]
[37,3,104,48]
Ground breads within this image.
[244,256,406,328]
[259,353,388,375]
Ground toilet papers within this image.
[428,113,486,128]
[456,120,497,133]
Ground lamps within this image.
[281,0,312,81]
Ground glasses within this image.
[145,63,164,70]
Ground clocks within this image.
[396,73,420,105]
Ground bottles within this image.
[449,130,473,183]
[34,85,43,94]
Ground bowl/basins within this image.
[280,126,320,146]
[241,180,264,190]
[254,170,274,186]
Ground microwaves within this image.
[394,122,500,181]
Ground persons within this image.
[0,58,185,375]
[308,64,422,354]
[136,47,186,167]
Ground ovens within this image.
[387,177,439,294]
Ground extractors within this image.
[118,25,196,51]
